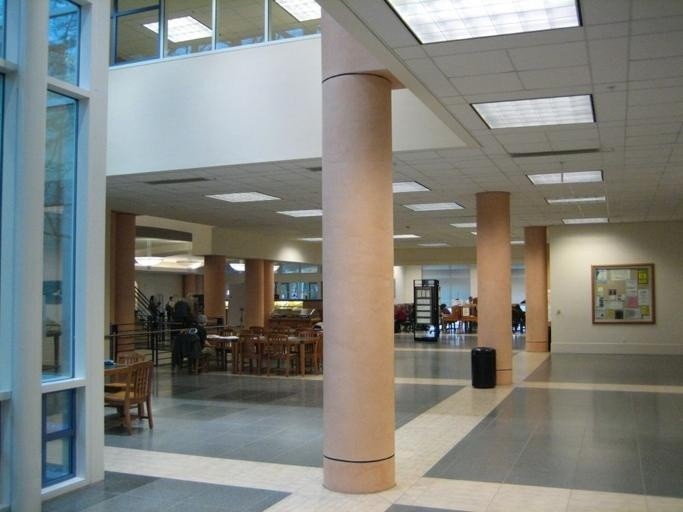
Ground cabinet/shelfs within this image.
[413,279,439,342]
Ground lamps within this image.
[229,259,245,272]
[176,243,202,270]
[134,240,163,268]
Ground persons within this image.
[520,300,526,334]
[439,296,477,335]
[394,302,414,333]
[135,292,215,375]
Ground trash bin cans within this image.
[471,347,496,388]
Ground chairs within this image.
[444,306,477,333]
[104,352,153,436]
[172,323,323,377]
